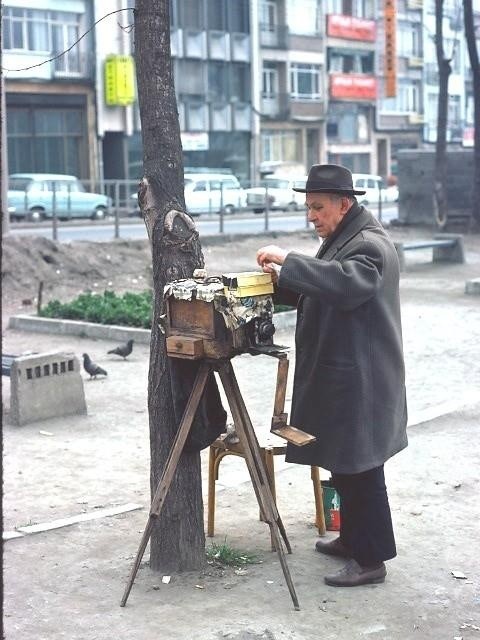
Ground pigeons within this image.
[82,352,108,380]
[107,338,135,362]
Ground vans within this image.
[245,175,309,212]
[131,173,246,216]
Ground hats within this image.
[292,165,365,195]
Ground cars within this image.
[352,172,400,206]
[8,172,111,222]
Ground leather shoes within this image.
[325,559,387,586]
[316,537,352,556]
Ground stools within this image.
[206,414,332,548]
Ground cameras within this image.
[246,310,276,346]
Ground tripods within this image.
[119,357,301,614]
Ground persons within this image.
[257,164,408,587]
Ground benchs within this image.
[391,230,465,267]
[1,350,88,424]
